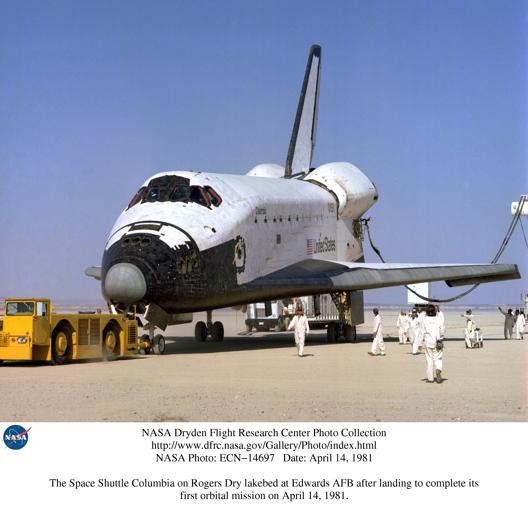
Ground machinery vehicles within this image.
[0,297,144,367]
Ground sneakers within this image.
[436,369,442,383]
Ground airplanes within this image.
[83,40,524,355]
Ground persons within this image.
[461,309,476,349]
[287,308,309,357]
[368,308,386,356]
[396,304,446,383]
[498,307,525,340]
